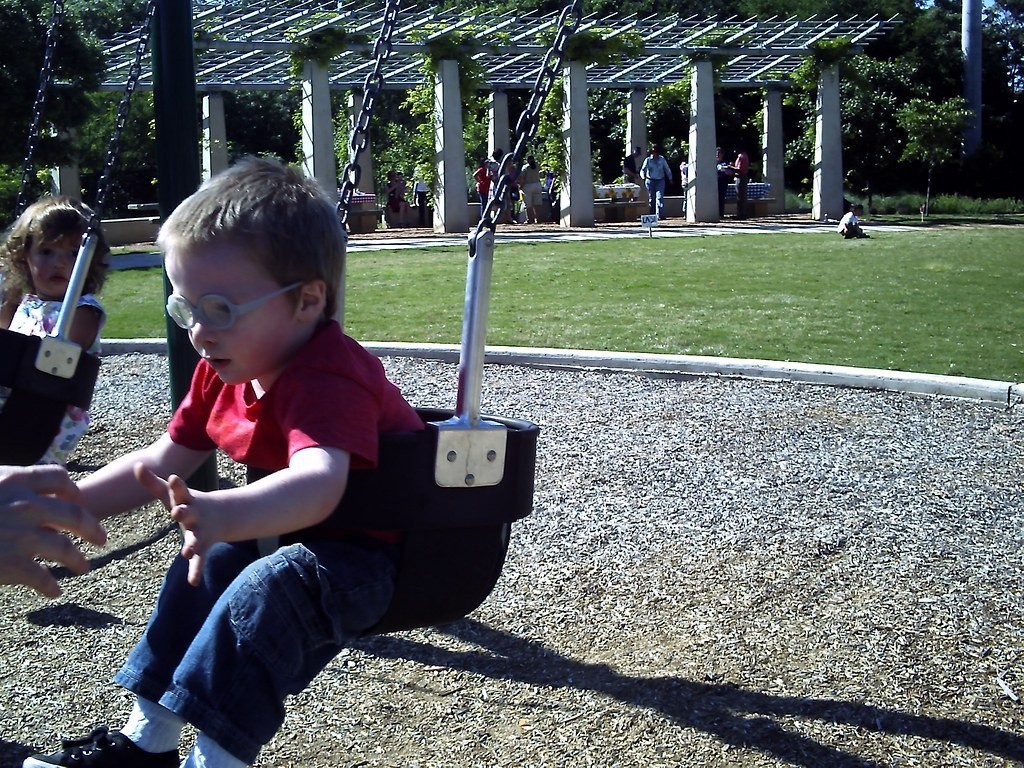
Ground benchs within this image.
[149,217,160,224]
[724,198,776,203]
[593,201,647,206]
[340,209,385,217]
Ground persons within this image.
[640,143,673,220]
[0,461,113,603]
[519,155,545,223]
[471,148,521,223]
[837,203,873,240]
[679,149,688,220]
[20,153,450,768]
[385,169,410,229]
[412,156,434,228]
[714,143,751,220]
[546,151,560,223]
[0,199,116,470]
[622,146,642,185]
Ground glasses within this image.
[165,279,307,329]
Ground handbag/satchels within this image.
[510,187,519,200]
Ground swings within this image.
[231,0,588,639]
[1,0,158,468]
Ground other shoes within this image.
[730,216,746,220]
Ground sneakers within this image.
[23,727,180,768]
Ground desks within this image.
[338,193,377,232]
[127,204,159,211]
[594,184,641,198]
[725,182,772,216]
